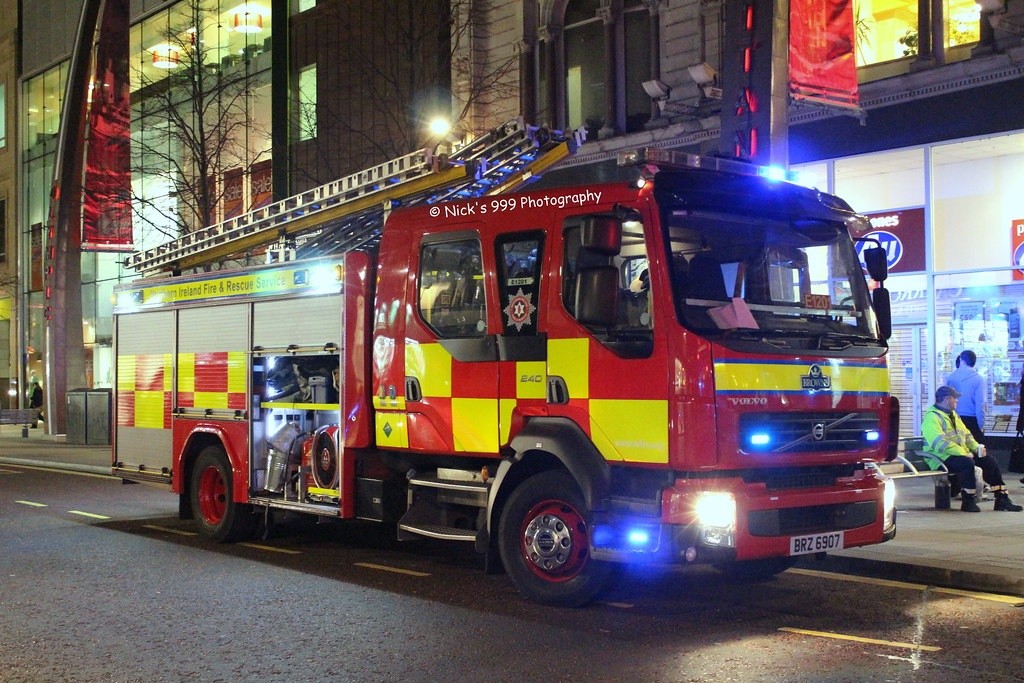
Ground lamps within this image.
[152,48,178,67]
[640,79,698,116]
[687,62,723,99]
[234,12,262,32]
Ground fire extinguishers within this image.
[284,428,317,491]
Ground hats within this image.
[936,386,962,396]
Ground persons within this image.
[1016,362,1024,485]
[29,382,45,429]
[946,350,990,493]
[920,386,1024,512]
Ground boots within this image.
[961,490,980,512]
[994,490,1023,511]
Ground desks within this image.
[203,36,272,76]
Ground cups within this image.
[977,445,985,457]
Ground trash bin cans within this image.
[87,388,112,445]
[65,388,90,445]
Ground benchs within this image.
[875,434,987,508]
[0,409,40,438]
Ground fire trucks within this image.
[112,119,903,606]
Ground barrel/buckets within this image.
[263,448,288,493]
[266,422,298,452]
